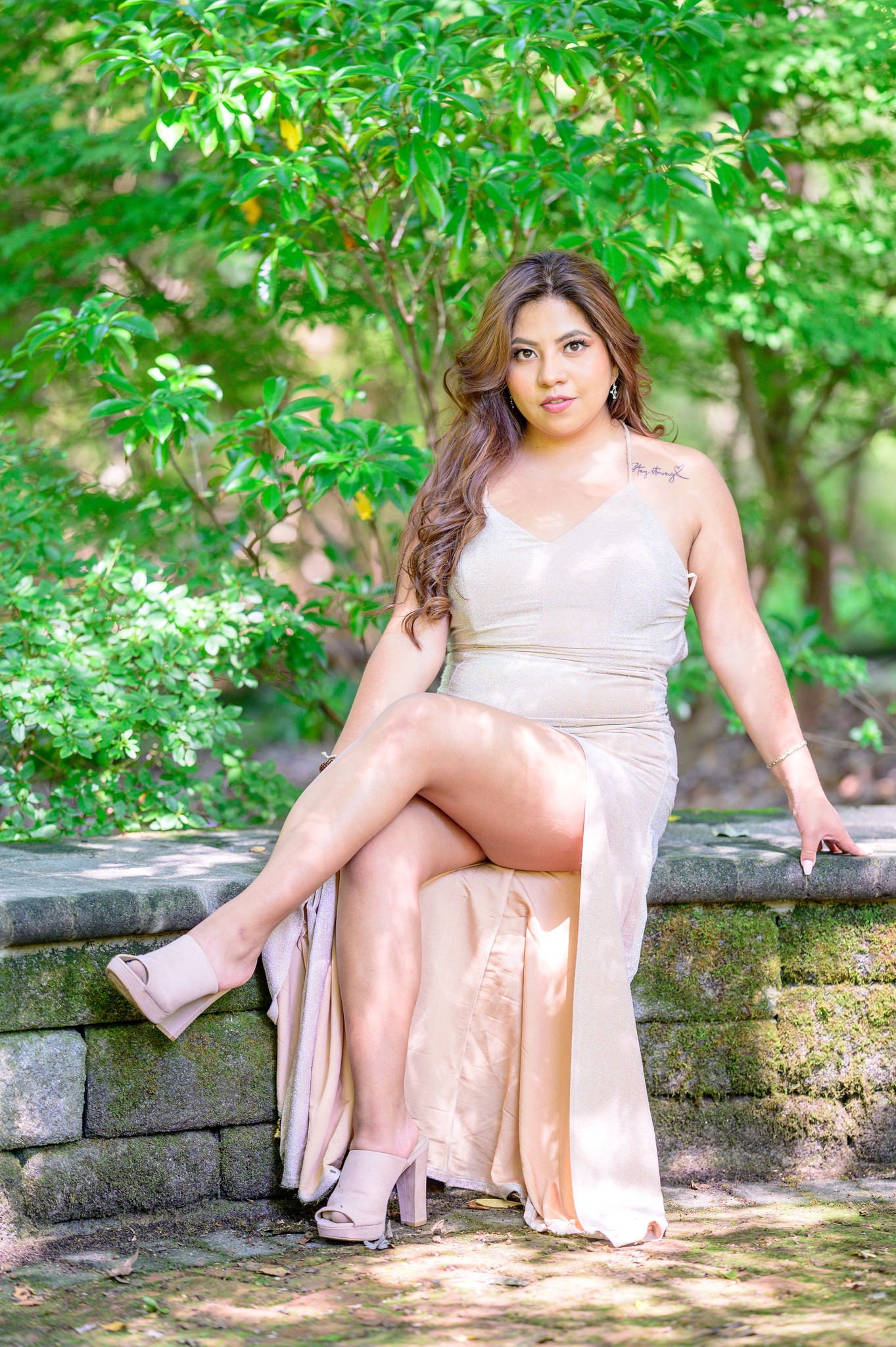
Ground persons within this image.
[105,249,874,1245]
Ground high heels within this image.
[106,933,231,1044]
[314,1135,431,1240]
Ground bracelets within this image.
[765,739,807,769]
[321,751,338,761]
[319,760,333,773]
